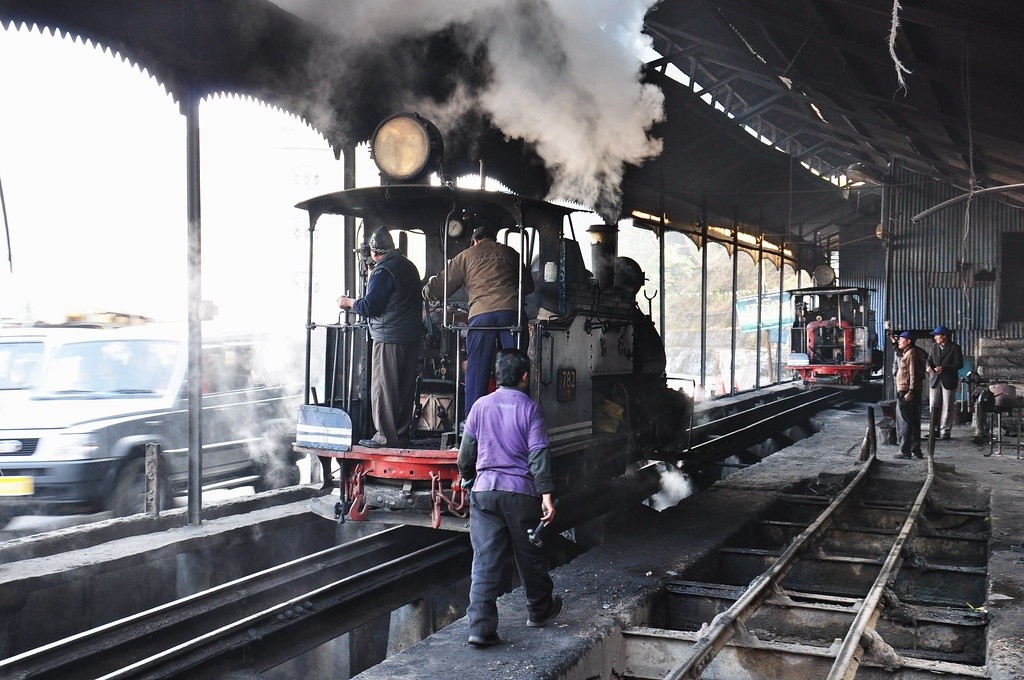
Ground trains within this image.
[771,281,883,393]
[279,110,700,565]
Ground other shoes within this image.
[912,450,923,459]
[452,421,464,432]
[923,431,940,438]
[358,439,384,448]
[942,432,950,440]
[893,452,911,459]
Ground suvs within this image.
[0,310,316,530]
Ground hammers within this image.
[528,499,562,547]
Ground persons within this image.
[886,330,929,458]
[456,348,562,642]
[338,225,421,446]
[422,226,535,431]
[925,326,962,438]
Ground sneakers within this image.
[467,630,501,646]
[526,592,563,628]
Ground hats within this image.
[469,225,495,246]
[368,225,395,254]
[929,326,948,336]
[894,331,917,340]
